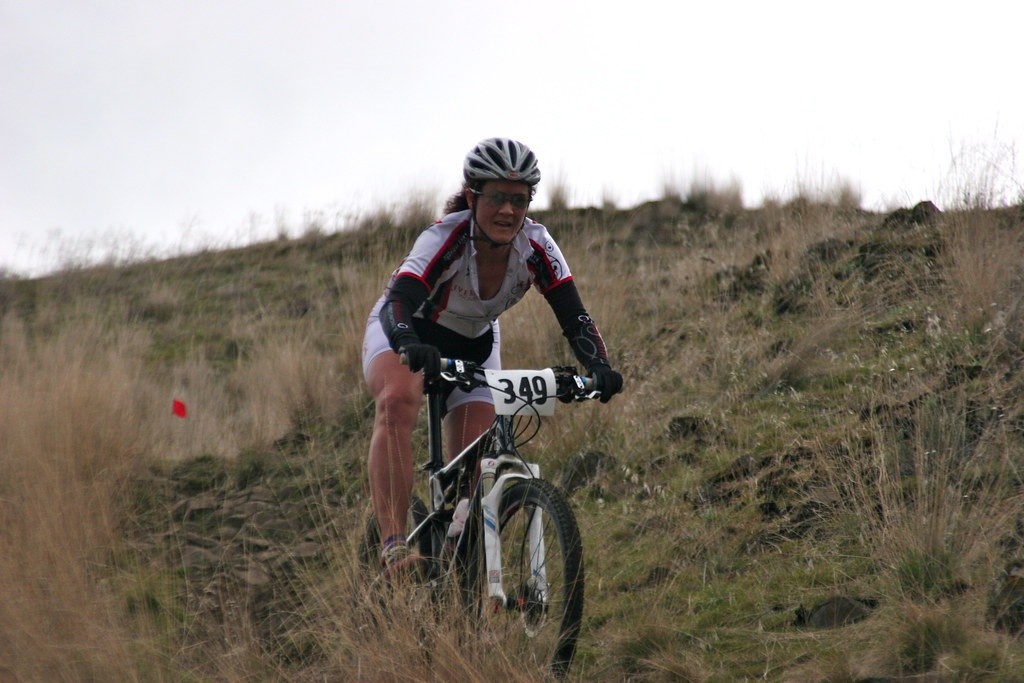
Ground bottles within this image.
[439,499,469,553]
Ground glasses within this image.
[469,185,533,210]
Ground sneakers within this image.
[379,541,428,585]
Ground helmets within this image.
[463,137,542,189]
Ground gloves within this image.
[586,366,623,405]
[399,342,441,378]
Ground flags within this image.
[172,400,186,418]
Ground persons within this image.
[363,137,624,589]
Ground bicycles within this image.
[344,347,625,683]
